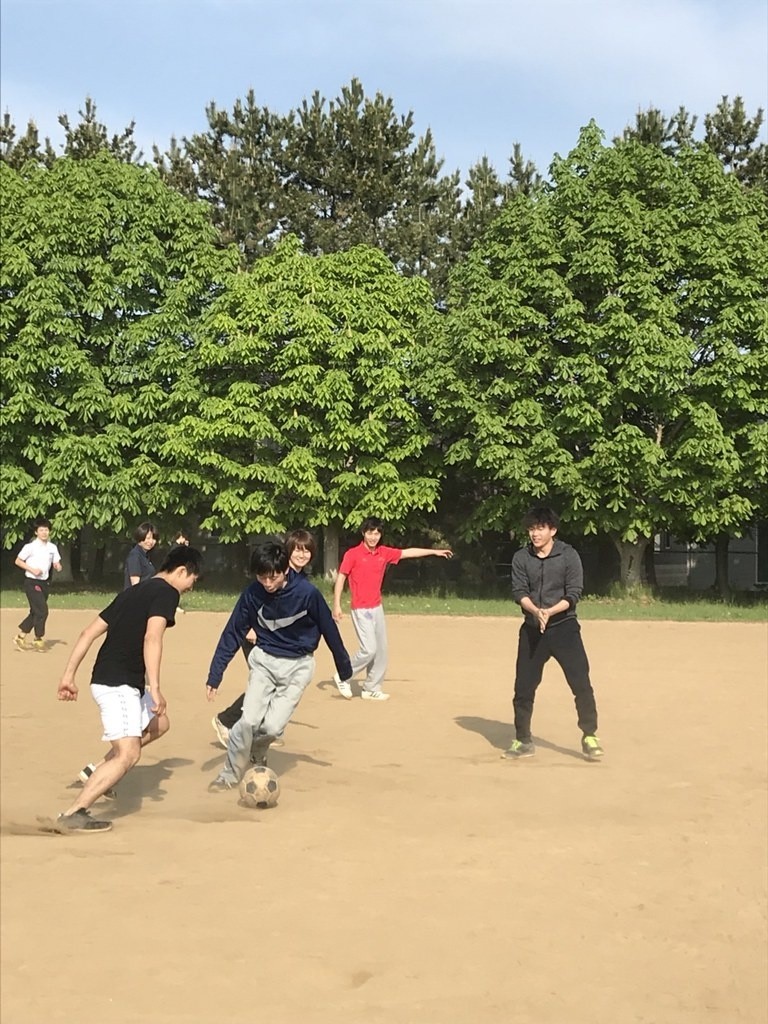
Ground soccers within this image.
[239,767,281,808]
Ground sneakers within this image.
[334,672,353,700]
[269,740,284,747]
[251,757,266,768]
[581,735,606,760]
[361,689,390,701]
[500,740,535,760]
[212,715,232,749]
[78,763,117,801]
[208,775,238,793]
[57,808,112,833]
[31,639,46,653]
[12,635,28,653]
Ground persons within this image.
[55,546,201,833]
[500,507,605,759]
[124,522,159,590]
[334,517,453,700]
[206,528,353,792]
[13,518,62,652]
